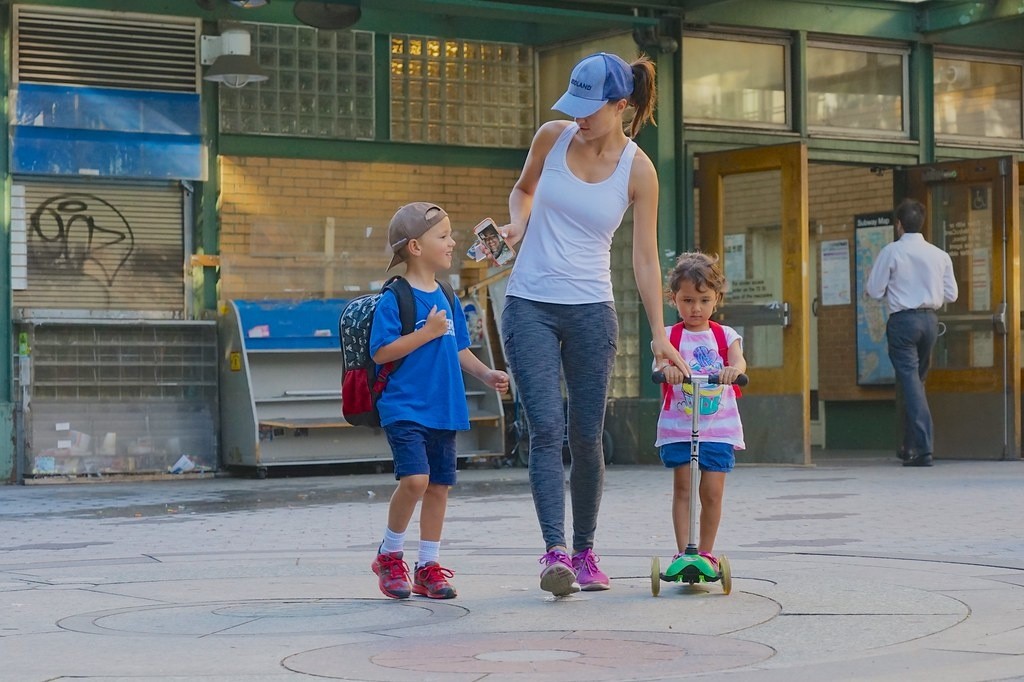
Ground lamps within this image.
[291,1,361,31]
[200,27,268,89]
[196,0,270,11]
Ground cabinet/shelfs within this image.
[219,296,506,480]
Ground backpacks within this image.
[339,277,455,428]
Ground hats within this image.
[385,202,448,272]
[551,52,634,118]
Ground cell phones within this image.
[473,217,516,265]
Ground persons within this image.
[477,53,690,598]
[371,201,509,599]
[481,228,504,256]
[651,252,746,574]
[866,199,958,467]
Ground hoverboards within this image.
[648,371,750,597]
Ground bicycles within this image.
[493,364,614,470]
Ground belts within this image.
[899,307,935,313]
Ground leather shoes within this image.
[896,447,913,459]
[902,454,933,467]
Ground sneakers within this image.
[698,550,720,573]
[672,551,688,562]
[413,561,457,599]
[371,539,413,598]
[538,550,580,597]
[572,548,609,590]
